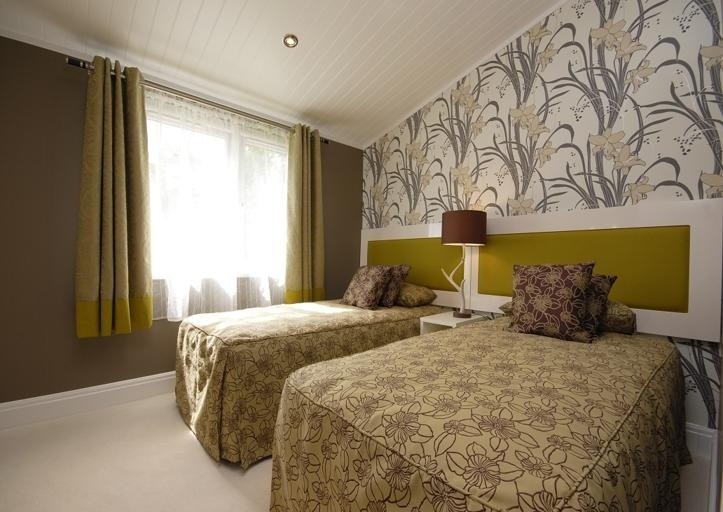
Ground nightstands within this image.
[418,310,481,336]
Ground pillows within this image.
[501,263,639,344]
[341,266,436,308]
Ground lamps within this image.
[437,209,488,318]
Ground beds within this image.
[267,317,693,512]
[175,299,454,474]
